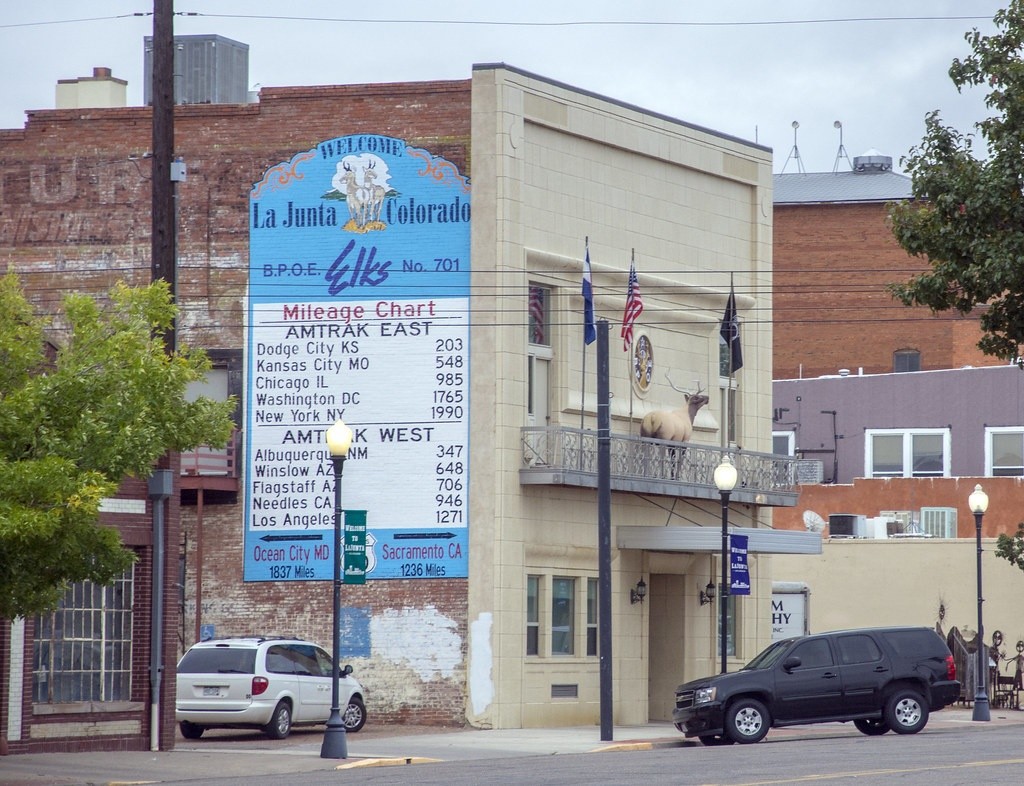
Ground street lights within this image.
[968,484,991,721]
[321,419,352,758]
[713,454,737,674]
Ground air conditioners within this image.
[792,459,824,485]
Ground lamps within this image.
[700,579,716,606]
[630,576,646,606]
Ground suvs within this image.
[175,636,367,739]
[672,626,961,746]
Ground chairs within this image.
[996,676,1015,708]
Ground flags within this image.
[582,246,596,345]
[719,287,743,373]
[529,287,544,343]
[621,260,643,352]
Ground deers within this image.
[639,365,710,481]
